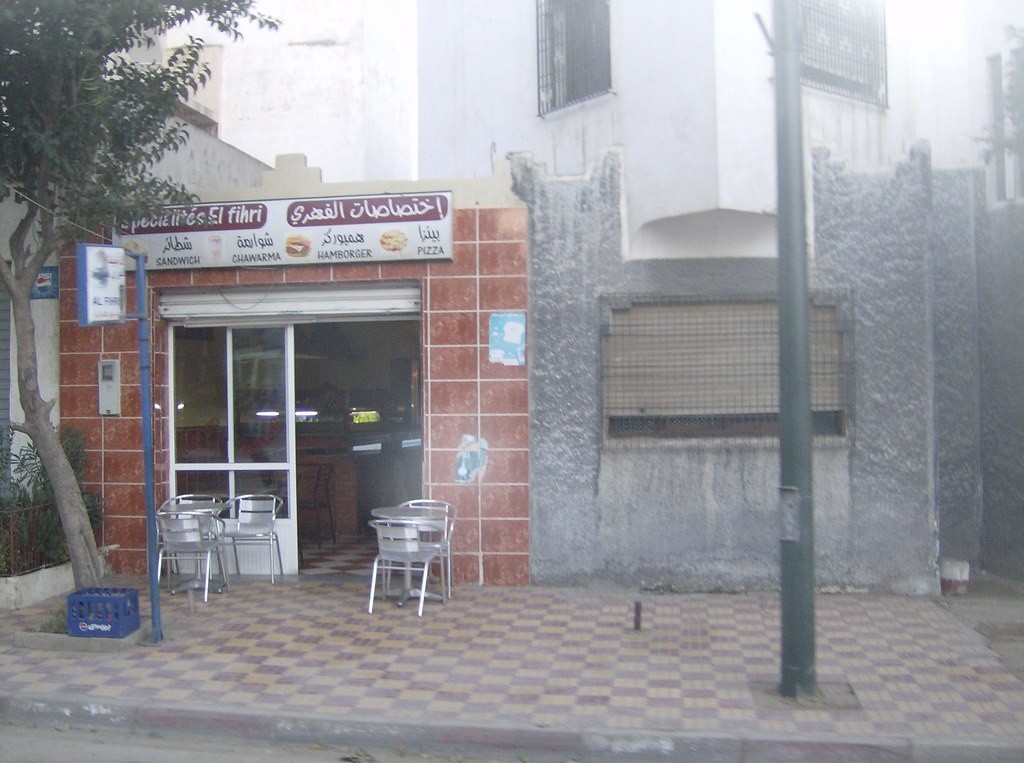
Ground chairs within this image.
[299,463,339,550]
[155,493,283,603]
[368,497,458,617]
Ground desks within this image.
[158,502,233,593]
[195,483,279,498]
[369,506,448,606]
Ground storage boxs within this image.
[67,586,140,640]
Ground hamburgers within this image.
[286,235,312,258]
[381,230,408,251]
